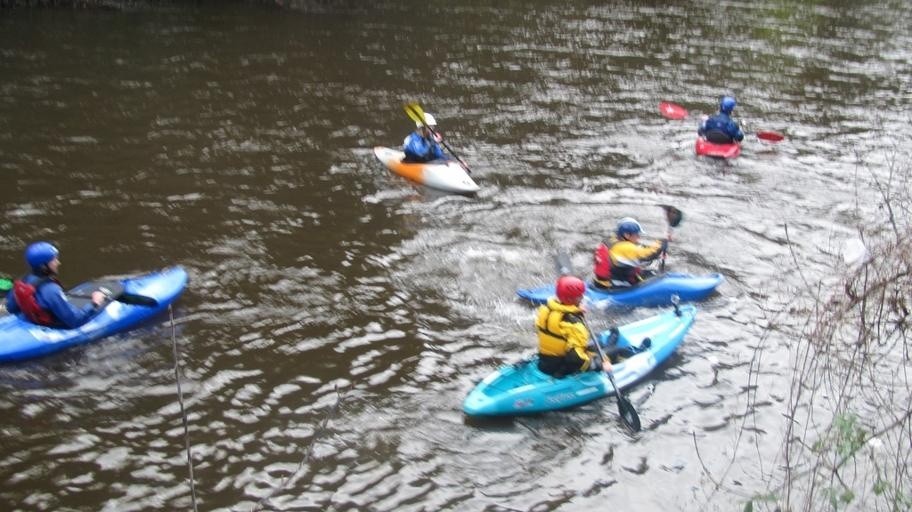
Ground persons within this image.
[402,112,449,163]
[591,217,672,289]
[6,241,112,330]
[537,275,651,379]
[698,96,744,144]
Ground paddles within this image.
[0,273,158,308]
[404,99,472,172]
[661,207,682,273]
[660,102,784,141]
[580,311,641,432]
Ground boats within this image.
[696,137,741,159]
[0,265,188,364]
[374,146,480,195]
[463,272,724,417]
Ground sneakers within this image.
[607,326,619,347]
[637,337,651,351]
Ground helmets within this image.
[25,242,60,268]
[721,97,736,112]
[415,112,437,129]
[556,275,586,305]
[617,217,646,235]
